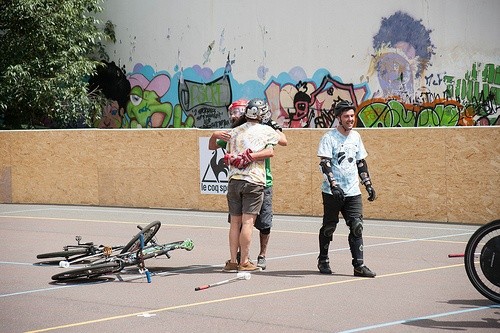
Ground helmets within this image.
[227,98,249,119]
[334,99,356,117]
[245,97,272,123]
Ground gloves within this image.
[366,185,376,201]
[232,148,254,168]
[223,152,236,165]
[330,183,345,199]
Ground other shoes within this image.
[353,265,376,277]
[317,258,332,273]
[257,254,266,269]
[223,259,238,272]
[237,261,262,273]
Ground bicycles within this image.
[463,219,500,304]
[36,221,194,287]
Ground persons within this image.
[317,100,376,278]
[224,98,287,272]
[209,101,274,269]
